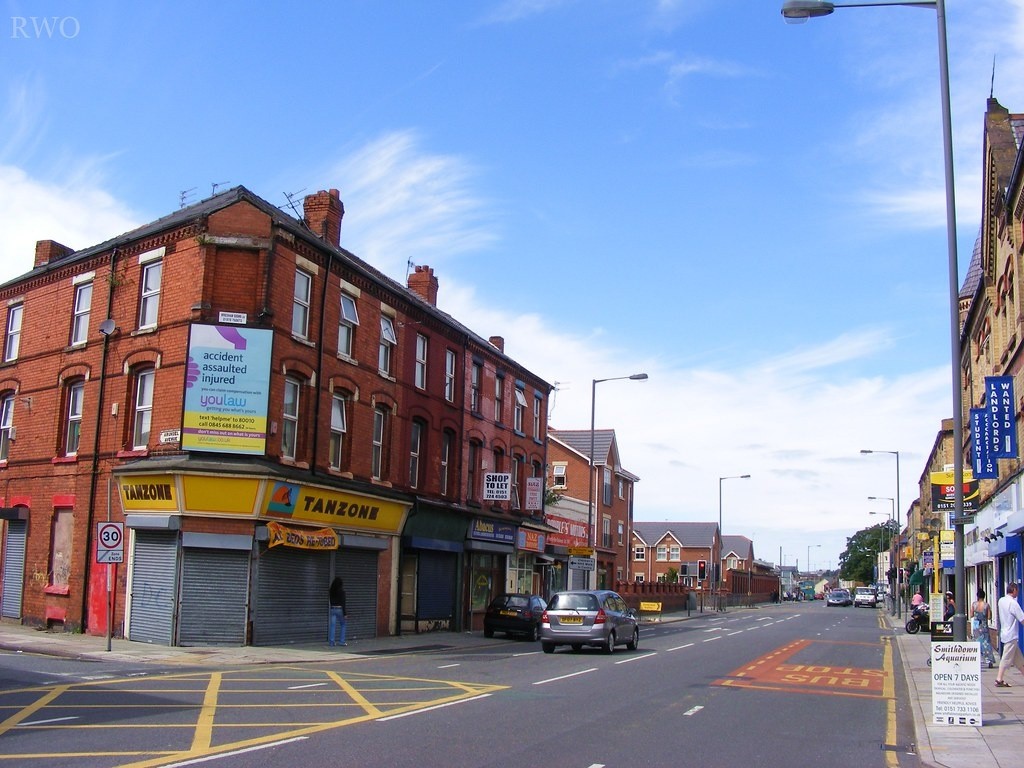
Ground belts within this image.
[331,607,342,609]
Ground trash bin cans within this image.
[930,621,953,641]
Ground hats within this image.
[945,591,953,596]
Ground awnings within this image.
[909,569,924,585]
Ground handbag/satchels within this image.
[910,601,914,610]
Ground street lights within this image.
[867,496,895,617]
[585,372,648,590]
[869,511,890,549]
[859,449,900,620]
[807,544,821,580]
[717,473,750,610]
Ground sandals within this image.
[994,680,1012,687]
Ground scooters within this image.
[905,603,930,634]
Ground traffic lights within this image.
[899,568,904,583]
[698,559,706,580]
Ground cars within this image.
[826,586,888,608]
[540,588,639,654]
[483,592,548,643]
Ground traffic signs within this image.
[569,557,595,571]
[568,545,594,556]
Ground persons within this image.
[329,578,348,647]
[944,590,956,626]
[912,591,923,609]
[994,582,1024,687]
[970,589,996,668]
[770,589,778,604]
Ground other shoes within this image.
[989,662,993,668]
[340,642,347,646]
